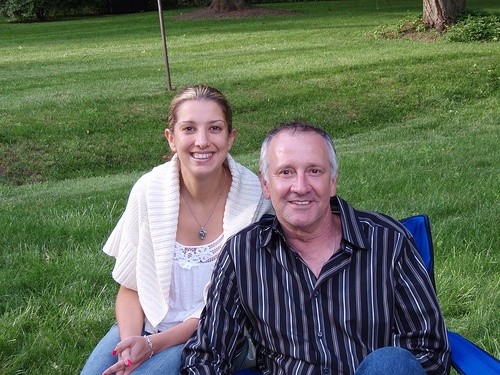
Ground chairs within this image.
[397,214,500,374]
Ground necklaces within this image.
[179,179,229,239]
[144,335,154,360]
[324,213,337,256]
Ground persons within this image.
[79,84,277,375]
[177,117,451,375]
